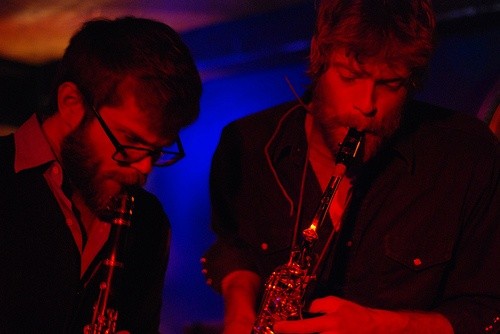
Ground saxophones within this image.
[85,188,137,334]
[243,128,368,334]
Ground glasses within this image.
[81,97,186,169]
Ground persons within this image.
[207,1,500,334]
[1,17,203,334]
